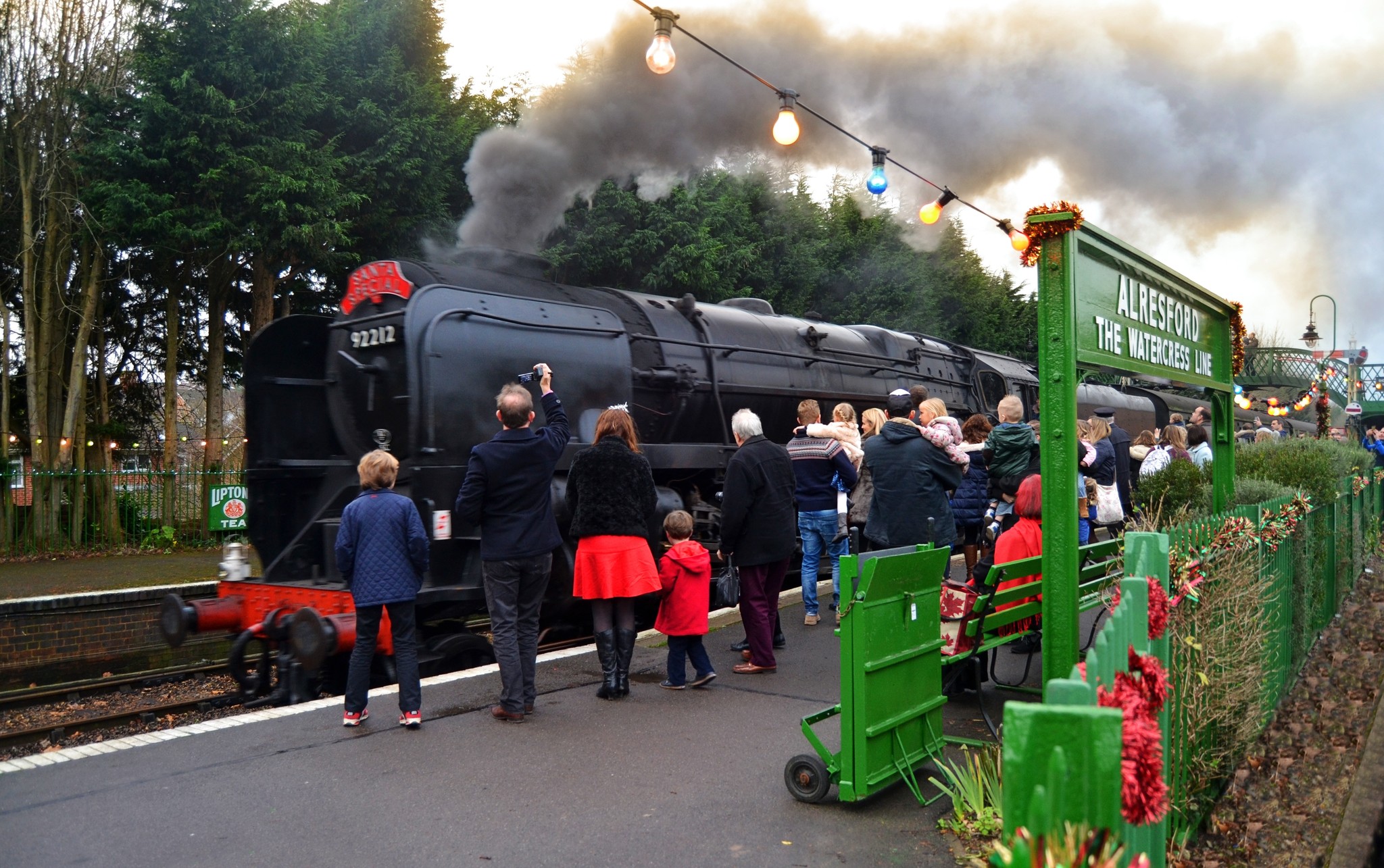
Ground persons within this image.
[333,448,429,726]
[654,510,717,689]
[730,610,785,651]
[1362,426,1384,467]
[1077,416,1116,582]
[1234,416,1287,445]
[456,362,571,722]
[563,401,662,700]
[848,408,888,552]
[962,473,1042,682]
[793,403,865,544]
[1328,427,1348,441]
[1243,332,1258,376]
[949,414,993,582]
[786,399,859,625]
[862,385,970,578]
[1093,405,1212,540]
[987,418,1041,530]
[717,409,797,674]
[982,391,1039,540]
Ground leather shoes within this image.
[733,661,777,674]
[772,633,785,647]
[730,639,752,651]
[742,649,752,660]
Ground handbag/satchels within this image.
[1092,482,1124,525]
[716,553,740,608]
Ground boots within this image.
[980,545,991,558]
[846,497,855,509]
[965,543,978,583]
[594,628,620,700]
[1078,498,1089,518]
[618,628,637,698]
[831,512,848,544]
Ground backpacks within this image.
[1139,445,1175,482]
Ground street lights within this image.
[1300,295,1337,438]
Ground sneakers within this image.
[400,709,421,725]
[659,678,685,689]
[805,613,820,625]
[835,614,840,623]
[343,709,368,725]
[986,523,998,541]
[688,671,717,686]
[984,510,995,527]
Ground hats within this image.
[887,389,914,416]
[1094,407,1114,418]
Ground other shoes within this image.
[524,705,532,714]
[492,705,524,723]
[1002,631,1042,654]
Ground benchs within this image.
[941,536,1125,752]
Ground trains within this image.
[158,258,1344,718]
